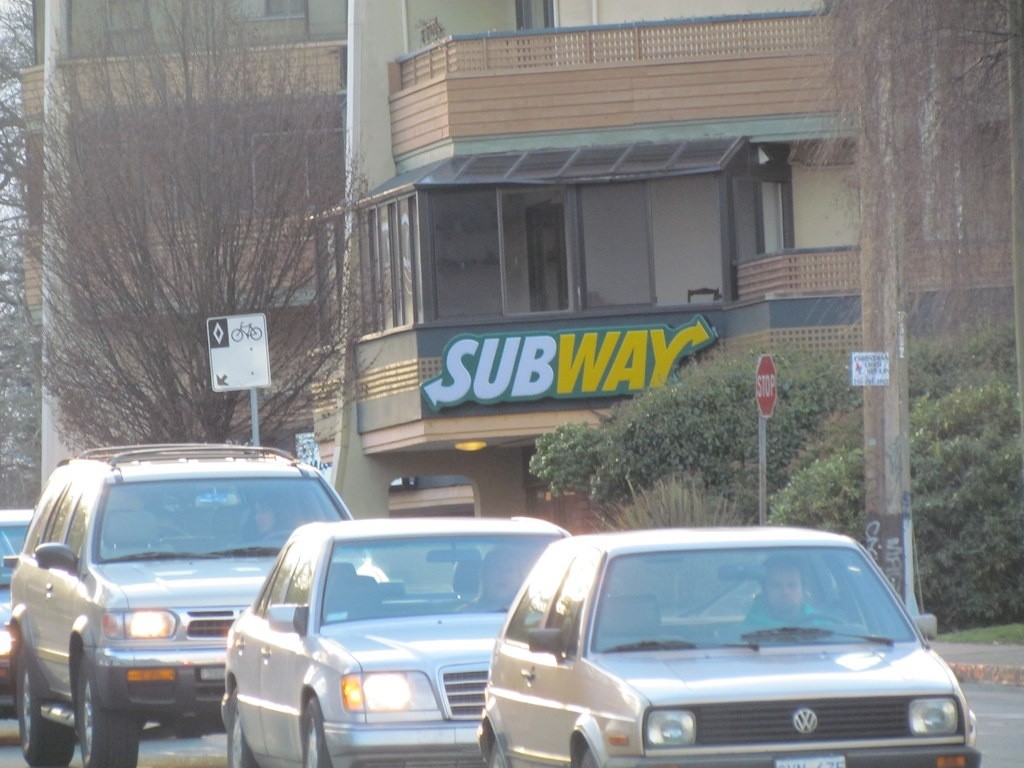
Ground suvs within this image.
[8,443,368,768]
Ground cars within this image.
[221,517,572,768]
[478,524,984,768]
[0,506,34,718]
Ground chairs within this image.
[596,593,665,643]
[326,575,382,614]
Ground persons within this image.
[742,550,846,634]
[457,549,523,614]
[246,494,297,538]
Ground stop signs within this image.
[755,354,777,418]
